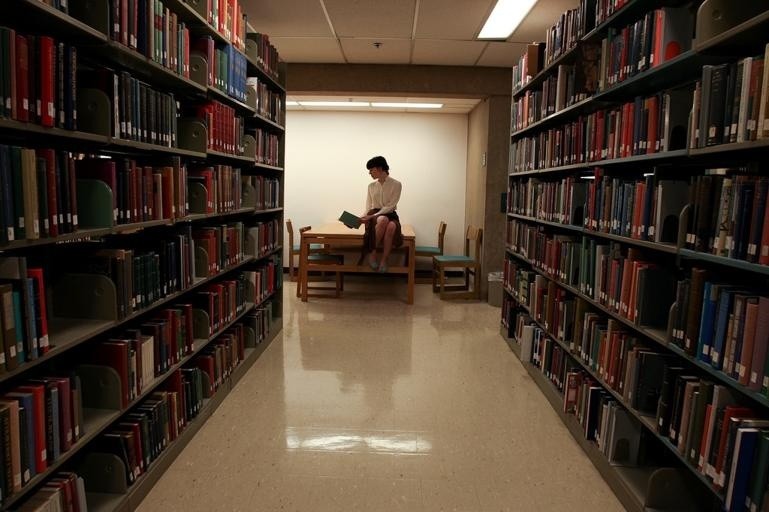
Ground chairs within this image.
[286,218,343,299]
[406,220,483,301]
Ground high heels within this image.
[369,253,386,273]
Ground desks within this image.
[300,223,415,304]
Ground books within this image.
[0,0,286,512]
[336,212,361,229]
[500,0,768,511]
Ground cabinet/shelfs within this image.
[497,0,769,511]
[0,2,287,511]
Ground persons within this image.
[356,156,402,274]
